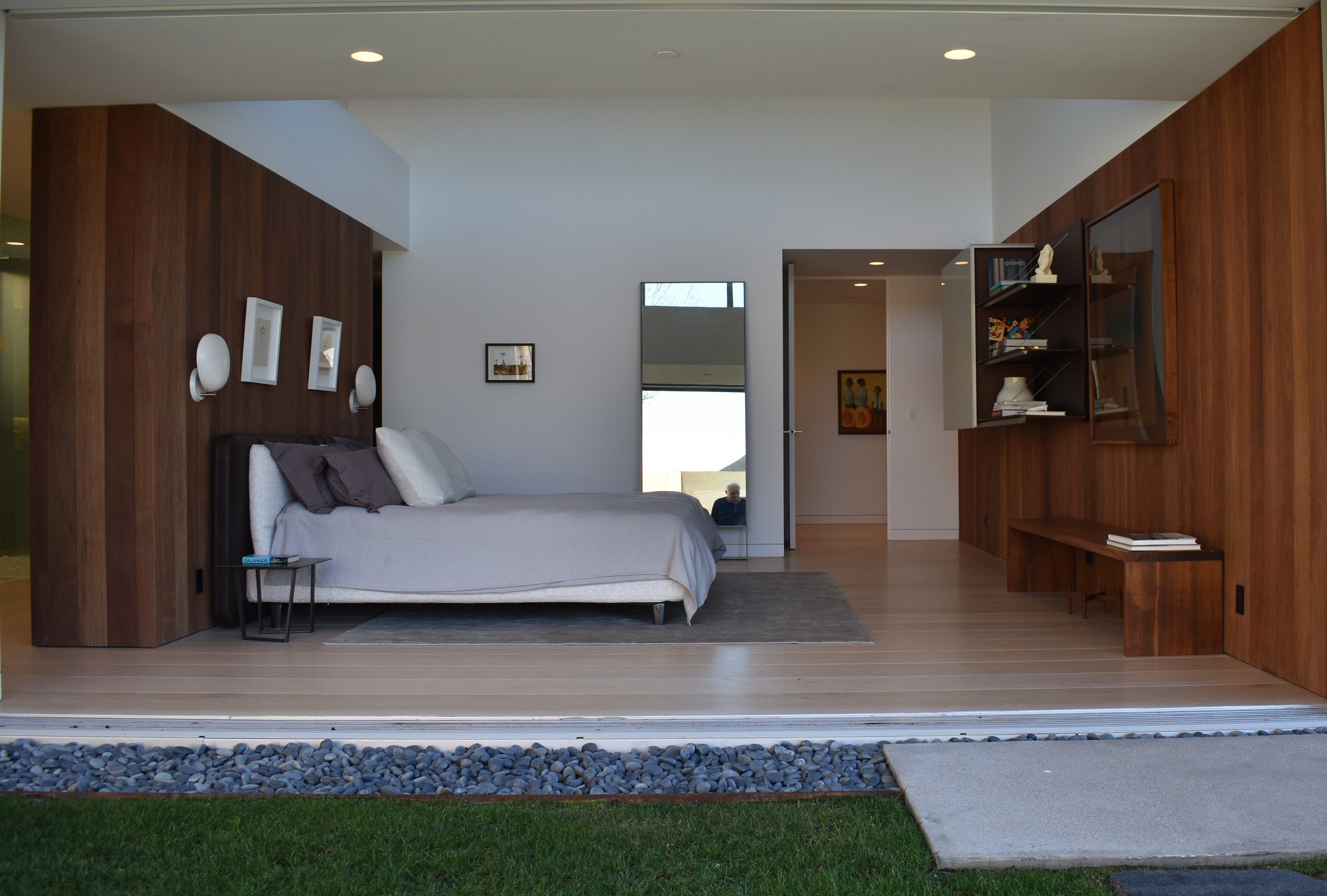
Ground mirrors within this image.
[637,279,750,562]
[1082,177,1182,450]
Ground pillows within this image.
[258,427,478,516]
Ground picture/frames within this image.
[484,341,535,384]
[307,314,343,392]
[241,295,284,385]
[835,368,888,435]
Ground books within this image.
[1108,531,1199,547]
[988,337,1048,350]
[987,257,1030,299]
[1106,539,1202,552]
[990,400,1066,418]
[988,347,1048,358]
[242,553,300,565]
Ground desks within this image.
[1004,515,1225,657]
[211,556,333,642]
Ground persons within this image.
[710,481,746,526]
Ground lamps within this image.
[347,362,378,416]
[187,332,231,405]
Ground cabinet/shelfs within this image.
[1085,251,1136,433]
[976,222,1085,430]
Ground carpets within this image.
[322,568,875,647]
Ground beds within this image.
[205,425,727,626]
